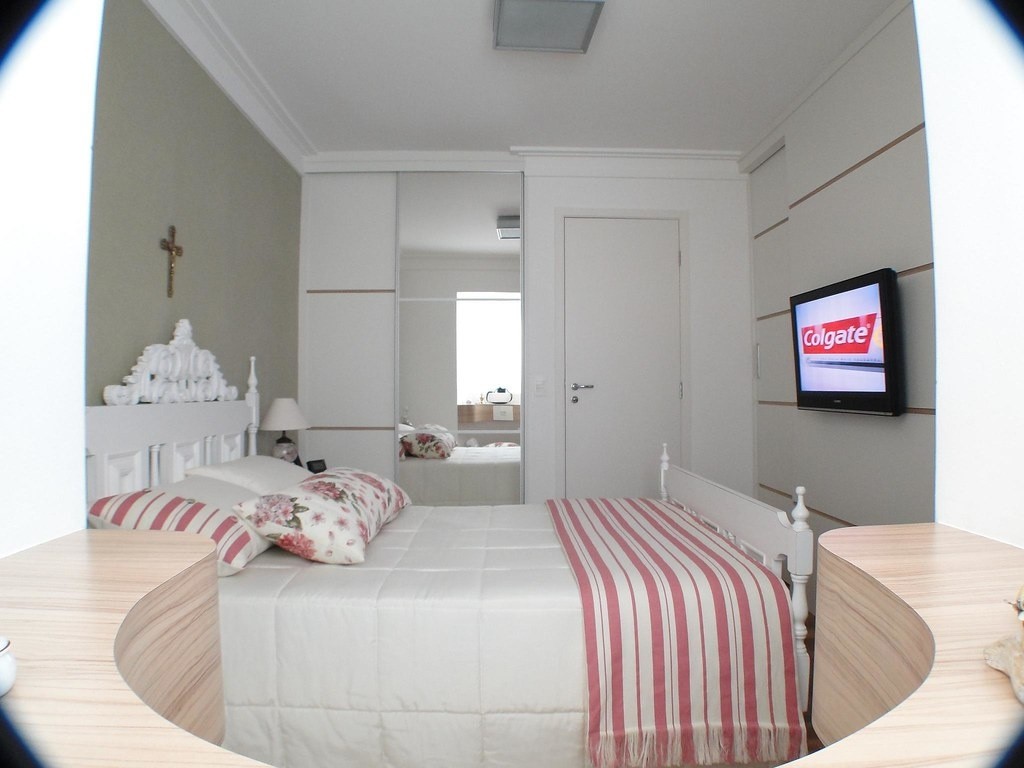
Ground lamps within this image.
[261,397,312,461]
[496,216,521,239]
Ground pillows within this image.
[397,424,415,433]
[407,423,457,457]
[397,434,408,461]
[233,465,412,564]
[485,442,518,447]
[186,453,314,495]
[167,475,257,510]
[89,484,273,577]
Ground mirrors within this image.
[394,170,525,507]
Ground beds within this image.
[86,321,819,768]
[399,447,521,506]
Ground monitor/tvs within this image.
[790,268,908,417]
